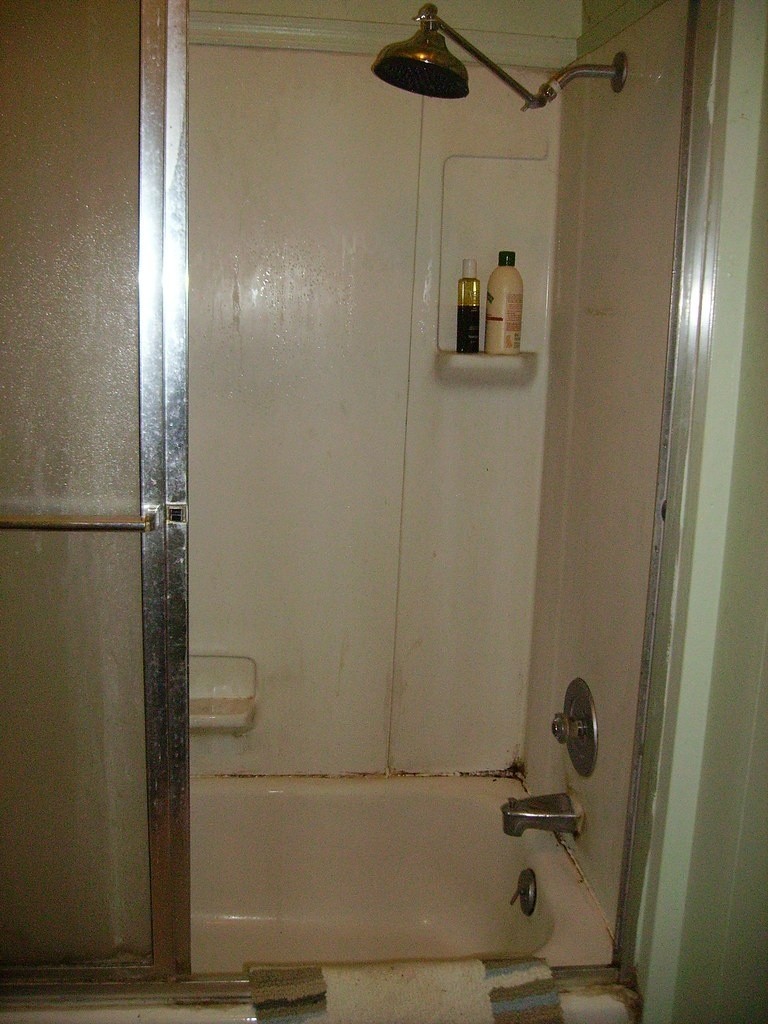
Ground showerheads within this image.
[370,2,469,100]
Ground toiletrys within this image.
[484,250,525,355]
[455,250,480,355]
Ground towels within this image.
[248,955,567,1024]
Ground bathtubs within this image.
[191,774,620,977]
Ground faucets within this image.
[500,792,577,838]
